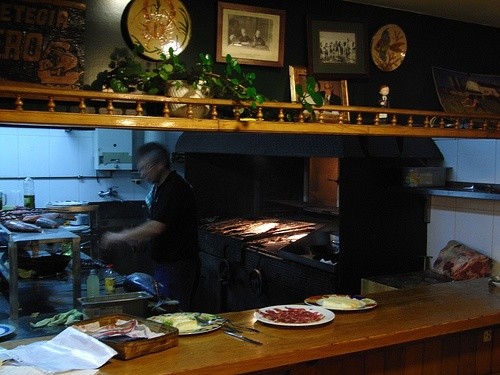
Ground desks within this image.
[0,278,500,375]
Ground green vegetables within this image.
[161,319,173,327]
[189,314,224,323]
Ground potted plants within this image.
[109,46,272,118]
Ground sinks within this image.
[123,217,144,227]
[98,218,131,233]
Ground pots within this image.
[24,246,92,277]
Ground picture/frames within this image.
[215,2,286,67]
[306,15,370,81]
[289,65,351,123]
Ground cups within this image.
[78,215,89,225]
[0,190,7,210]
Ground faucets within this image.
[98,188,124,204]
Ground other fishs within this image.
[22,213,74,224]
[35,218,58,228]
[3,220,41,233]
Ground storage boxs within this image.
[402,166,449,188]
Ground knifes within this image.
[224,330,263,346]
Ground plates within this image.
[0,324,16,337]
[304,294,377,310]
[148,312,225,335]
[127,0,191,60]
[254,305,336,326]
[371,24,407,72]
[46,200,88,208]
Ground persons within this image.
[315,81,341,114]
[378,84,390,123]
[319,38,355,59]
[229,27,265,46]
[100,143,202,313]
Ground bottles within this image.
[105,265,115,295]
[23,176,35,211]
[86,269,99,297]
